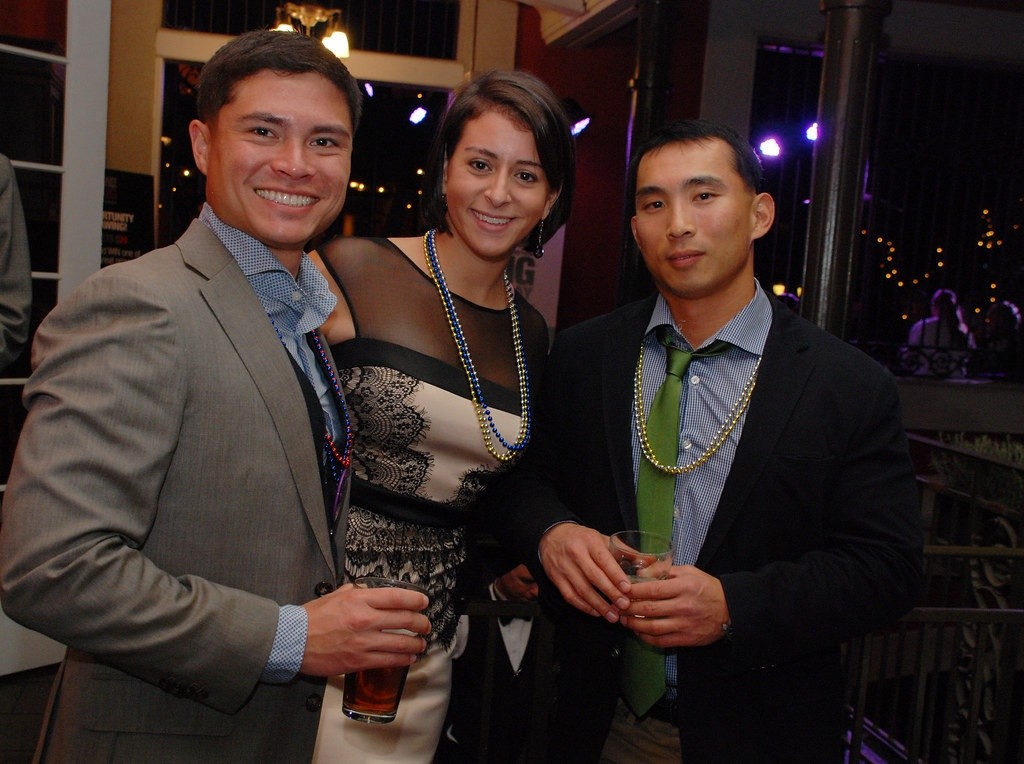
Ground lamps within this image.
[268,3,350,61]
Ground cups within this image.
[606,527,677,629]
[341,577,435,726]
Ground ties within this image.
[624,330,735,716]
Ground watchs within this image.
[722,620,737,643]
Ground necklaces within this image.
[263,306,355,522]
[632,337,763,474]
[424,229,531,461]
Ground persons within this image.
[909,289,975,379]
[985,300,1020,331]
[489,118,923,764]
[311,67,577,764]
[0,153,33,371]
[1,30,434,764]
[475,565,540,764]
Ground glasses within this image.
[322,465,339,575]
